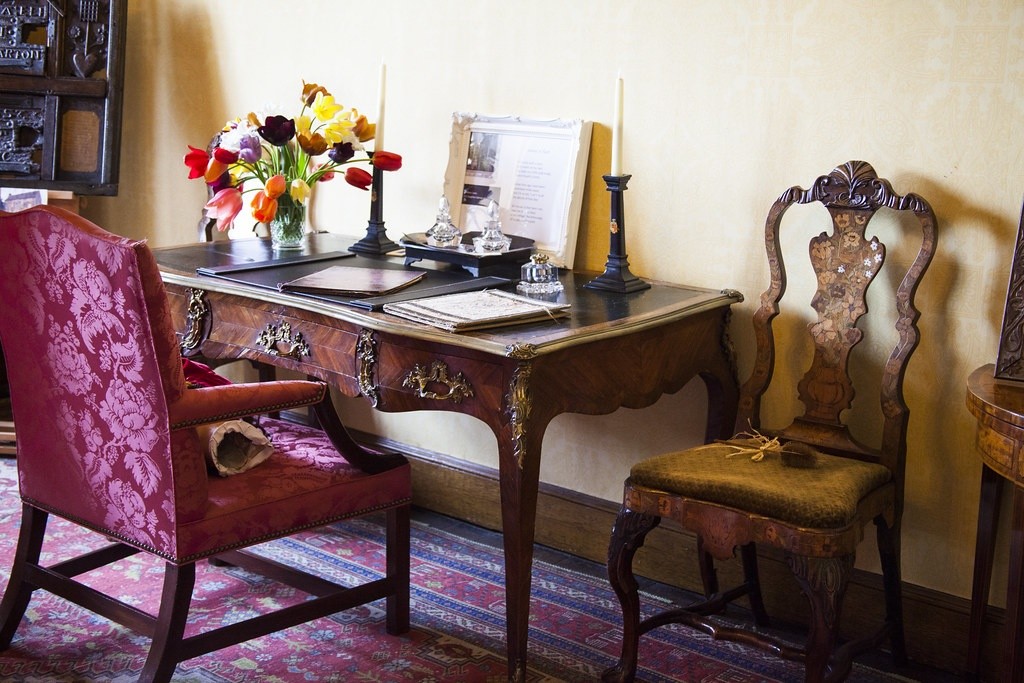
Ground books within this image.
[279,265,428,299]
[382,289,573,334]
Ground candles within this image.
[374,63,387,152]
[611,76,623,177]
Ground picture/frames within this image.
[443,111,592,269]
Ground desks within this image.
[151,233,740,683]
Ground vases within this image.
[270,207,305,250]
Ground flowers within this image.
[185,80,403,240]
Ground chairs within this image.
[600,161,939,683]
[0,206,411,683]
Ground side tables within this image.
[965,363,1024,683]
